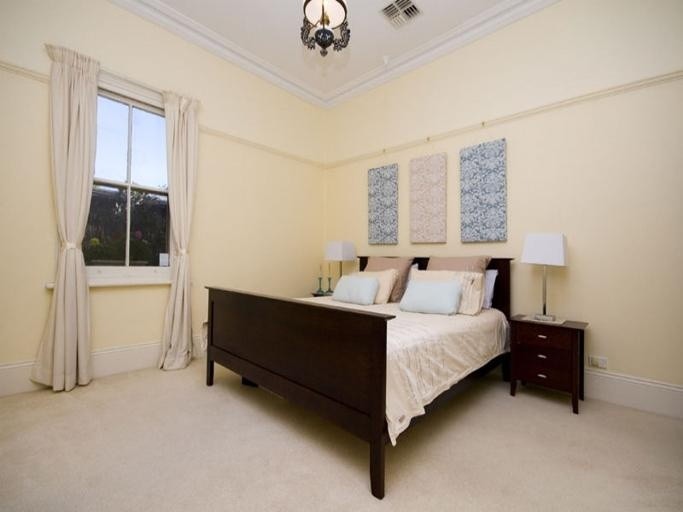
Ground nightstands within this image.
[508,312,589,415]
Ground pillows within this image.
[329,255,497,316]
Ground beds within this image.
[202,254,516,498]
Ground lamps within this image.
[300,0,349,60]
[520,231,567,322]
[303,241,358,296]
